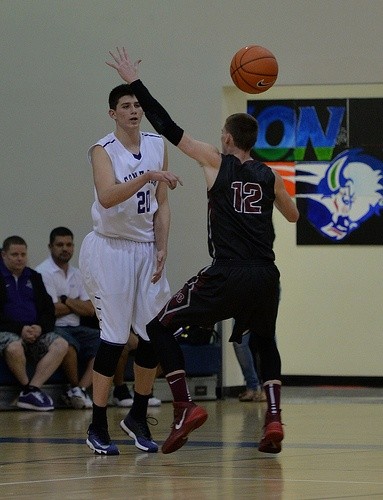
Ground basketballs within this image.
[230,46,280,94]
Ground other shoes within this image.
[239,388,254,401]
[253,388,267,401]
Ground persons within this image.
[113,370,161,407]
[105,45,299,454]
[35,226,100,407]
[0,236,67,410]
[79,83,184,456]
[232,319,266,403]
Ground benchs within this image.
[1,344,222,409]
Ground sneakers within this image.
[85,424,119,455]
[161,403,208,454]
[147,397,161,407]
[258,421,284,454]
[17,391,54,411]
[64,385,93,408]
[113,382,134,407]
[120,416,158,453]
